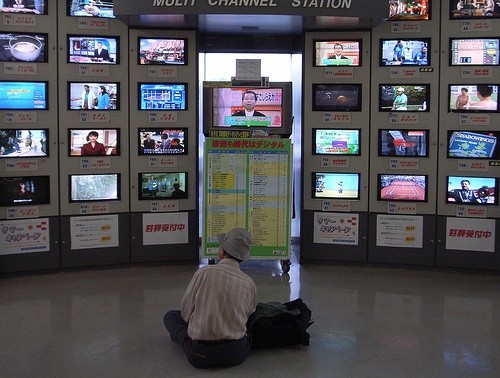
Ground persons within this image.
[81,131,106,155]
[393,40,403,61]
[327,44,348,59]
[391,87,407,110]
[94,41,109,60]
[17,182,32,195]
[74,41,80,50]
[231,91,267,127]
[170,184,185,197]
[84,0,102,16]
[144,133,183,152]
[456,88,470,110]
[11,0,27,8]
[149,175,158,196]
[413,46,427,62]
[470,84,497,110]
[82,84,110,109]
[163,228,258,369]
[457,0,494,16]
[448,180,477,202]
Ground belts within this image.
[199,339,234,346]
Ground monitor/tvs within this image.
[0,0,188,205]
[204,81,293,137]
[312,0,500,206]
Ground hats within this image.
[218,228,253,260]
